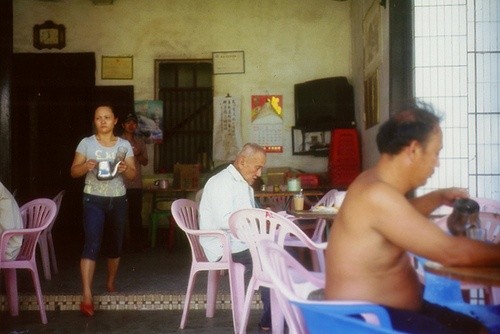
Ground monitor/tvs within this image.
[295,76,356,131]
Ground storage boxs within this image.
[267,168,290,185]
[173,162,201,187]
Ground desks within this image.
[253,192,324,197]
[423,261,500,288]
[131,188,199,246]
[286,209,338,220]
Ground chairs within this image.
[256,188,500,334]
[228,208,327,334]
[37,190,66,281]
[0,198,58,324]
[171,199,253,334]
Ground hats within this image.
[123,113,138,123]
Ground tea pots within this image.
[93,160,122,181]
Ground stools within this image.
[328,129,363,187]
[148,212,184,249]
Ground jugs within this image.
[445,200,481,235]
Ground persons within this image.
[324,107,500,334]
[197,143,290,334]
[0,181,23,263]
[70,103,137,317]
[118,111,148,258]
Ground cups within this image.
[291,194,304,211]
[468,226,487,244]
[154,178,170,190]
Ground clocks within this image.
[33,20,65,50]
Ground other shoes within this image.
[259,323,271,330]
[80,303,93,317]
